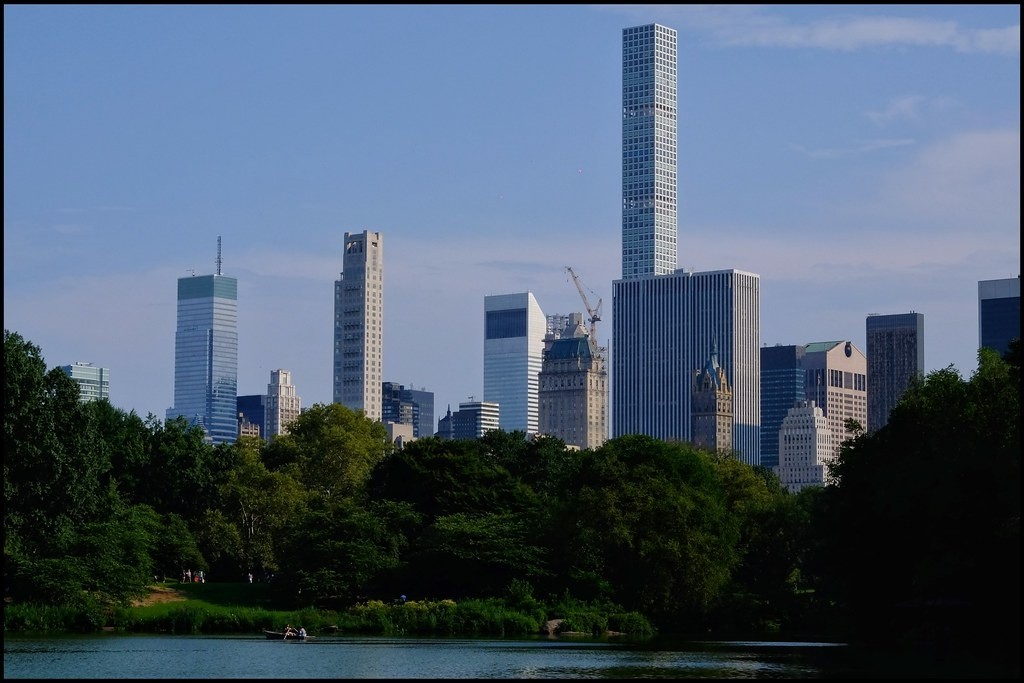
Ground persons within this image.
[401,593,407,604]
[248,573,253,583]
[298,626,306,636]
[163,575,167,583]
[181,569,204,583]
[283,623,296,636]
[154,575,158,582]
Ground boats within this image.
[262,628,315,641]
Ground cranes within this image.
[563,264,603,354]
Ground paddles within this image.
[283,629,290,639]
[294,628,298,633]
